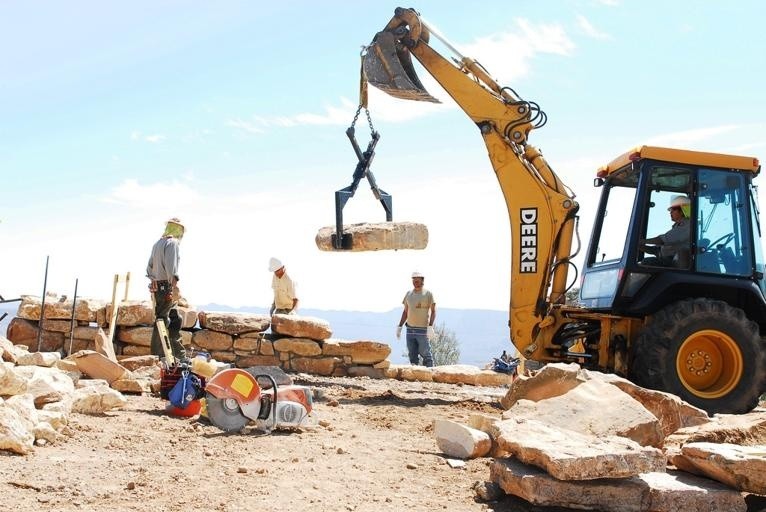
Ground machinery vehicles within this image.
[332,4,766,416]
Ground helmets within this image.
[268,257,285,271]
[165,217,186,229]
[409,272,425,278]
[667,195,691,211]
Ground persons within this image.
[640,196,691,266]
[396,272,436,367]
[145,218,192,363]
[269,258,299,318]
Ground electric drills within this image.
[205,368,312,435]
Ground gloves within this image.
[427,326,434,339]
[172,288,182,302]
[270,304,275,317]
[288,309,296,316]
[396,327,402,339]
